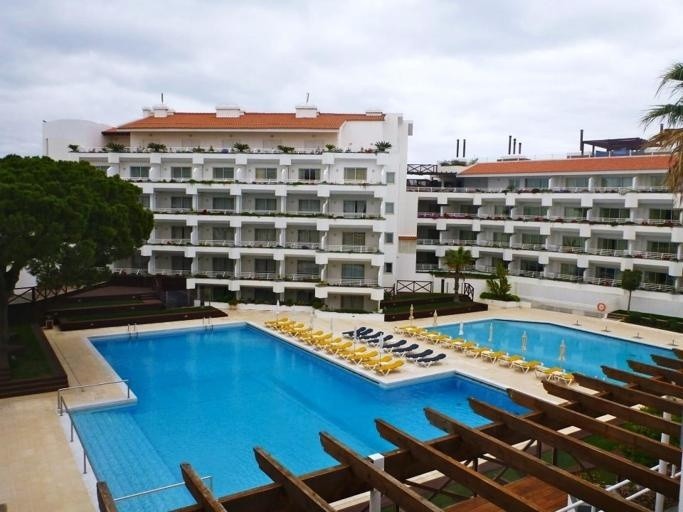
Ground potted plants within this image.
[375,141,392,152]
[277,145,294,153]
[105,141,126,153]
[233,142,249,152]
[147,143,167,152]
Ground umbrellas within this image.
[409,303,569,368]
[270,300,385,365]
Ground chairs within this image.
[265,314,576,387]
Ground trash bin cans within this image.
[46,320,54,329]
